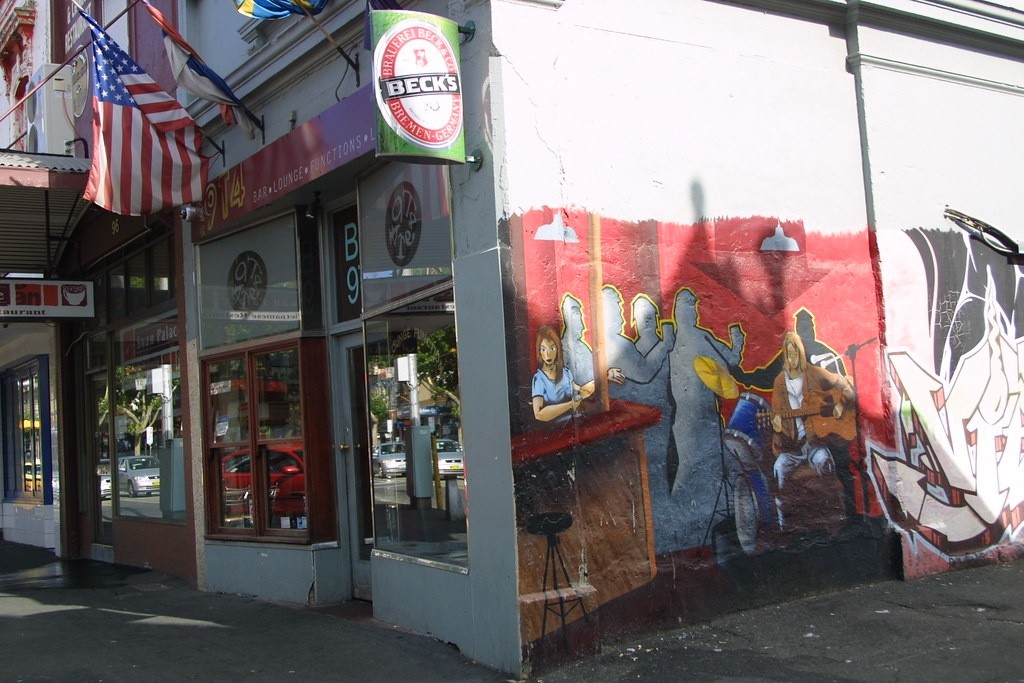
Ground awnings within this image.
[0,152,91,275]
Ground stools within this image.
[527,514,593,648]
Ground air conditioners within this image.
[25,64,89,159]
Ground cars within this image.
[24,463,42,491]
[99,455,161,499]
[431,439,463,479]
[220,443,305,515]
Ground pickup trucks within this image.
[372,442,406,478]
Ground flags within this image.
[141,0,254,141]
[233,0,328,20]
[78,7,209,216]
